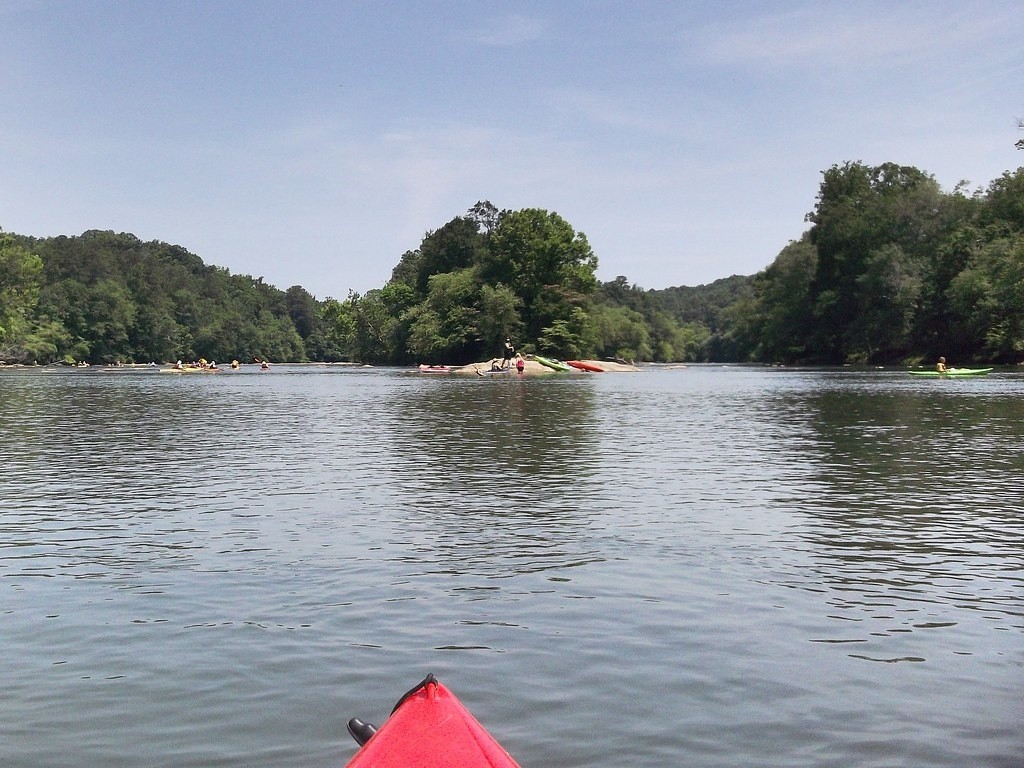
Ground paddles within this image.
[255,357,270,370]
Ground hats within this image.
[492,358,499,362]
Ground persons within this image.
[515,352,524,374]
[492,357,502,371]
[500,337,514,368]
[172,356,216,369]
[936,357,947,371]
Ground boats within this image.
[534,356,569,373]
[158,364,219,372]
[260,367,268,372]
[419,363,450,372]
[908,368,994,376]
[567,360,604,371]
[78,364,90,368]
[231,367,239,371]
